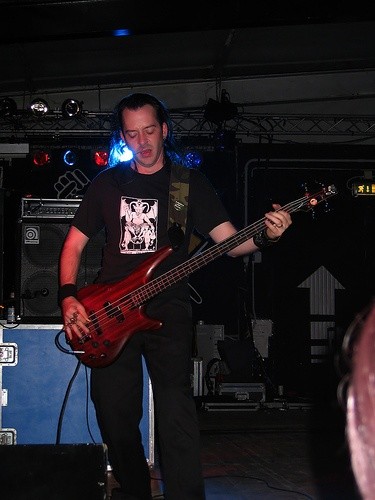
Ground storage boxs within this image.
[193,357,267,403]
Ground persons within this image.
[344,295,375,500]
[58,92,293,499]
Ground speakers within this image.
[0,443,110,500]
[14,218,105,325]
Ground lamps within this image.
[29,98,50,116]
[0,97,17,118]
[34,149,51,168]
[62,98,82,117]
[93,146,109,166]
[202,96,240,124]
[64,149,78,166]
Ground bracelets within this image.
[58,284,78,308]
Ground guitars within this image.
[67,179,339,368]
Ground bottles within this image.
[7,292,18,323]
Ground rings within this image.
[73,312,78,318]
[277,224,283,228]
[68,322,72,326]
[64,324,67,328]
[70,317,77,324]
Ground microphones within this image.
[134,150,140,155]
